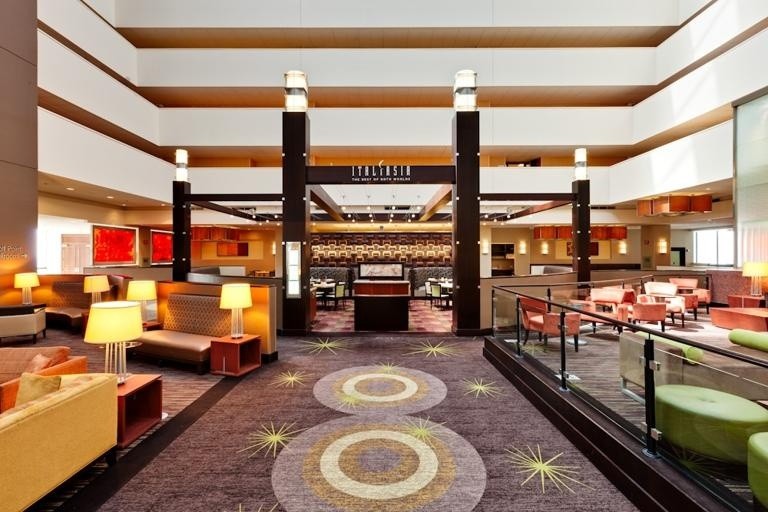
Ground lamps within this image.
[247,191,515,228]
[84,302,142,385]
[15,272,41,305]
[742,262,767,295]
[219,282,251,337]
[126,281,158,323]
[83,275,110,303]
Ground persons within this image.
[320,273,328,285]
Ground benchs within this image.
[135,293,233,374]
[35,281,118,328]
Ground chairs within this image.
[422,276,454,312]
[310,276,347,311]
[515,278,767,350]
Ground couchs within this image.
[1,373,120,510]
[0,304,47,343]
[0,346,86,410]
[615,326,768,407]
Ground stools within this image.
[655,383,768,465]
[748,431,767,509]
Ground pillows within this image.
[23,352,53,373]
[13,373,61,408]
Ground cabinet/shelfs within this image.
[82,312,90,335]
[116,373,163,445]
[211,334,262,376]
[143,321,160,328]
